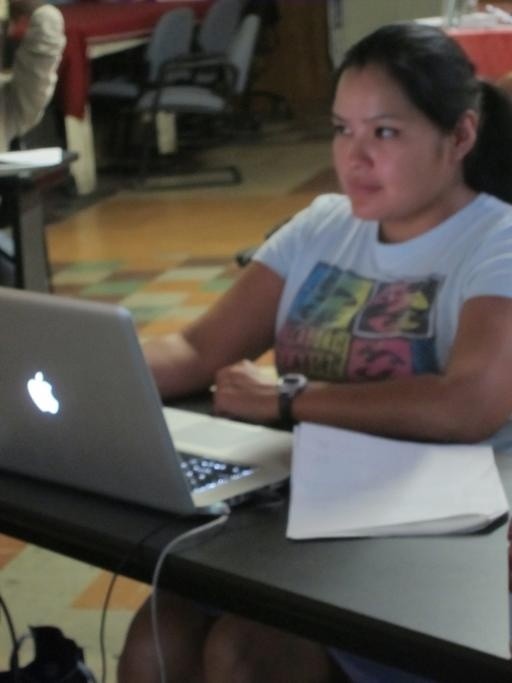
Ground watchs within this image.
[278,373,308,425]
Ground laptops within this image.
[0,285,294,519]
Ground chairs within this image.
[88,0,293,175]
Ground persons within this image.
[0,0,66,288]
[115,23,512,683]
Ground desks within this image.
[0,393,509,678]
